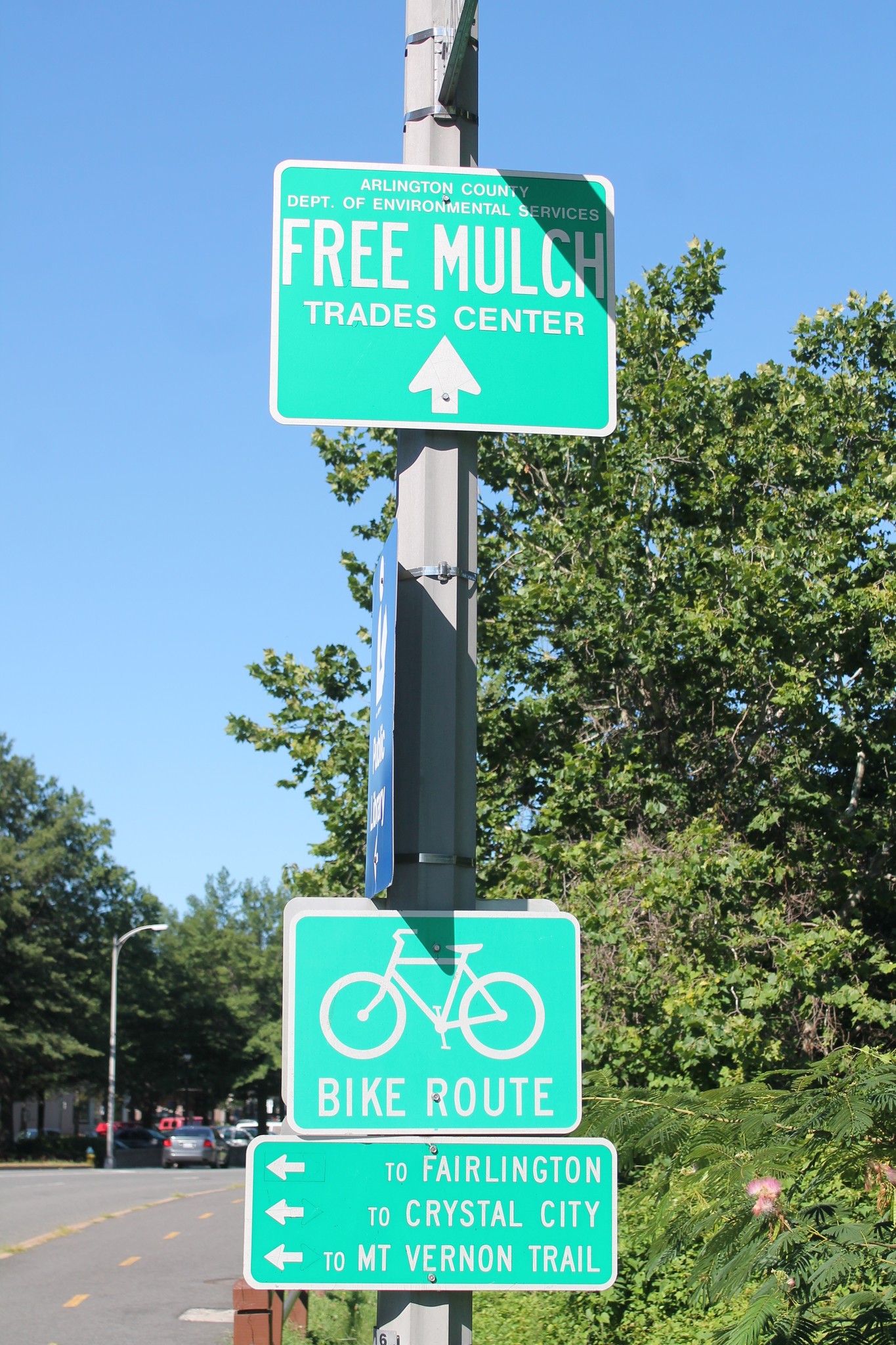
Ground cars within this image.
[12,1115,278,1159]
[161,1125,229,1169]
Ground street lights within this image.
[106,920,170,1168]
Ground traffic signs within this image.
[267,161,618,437]
[244,1136,616,1293]
